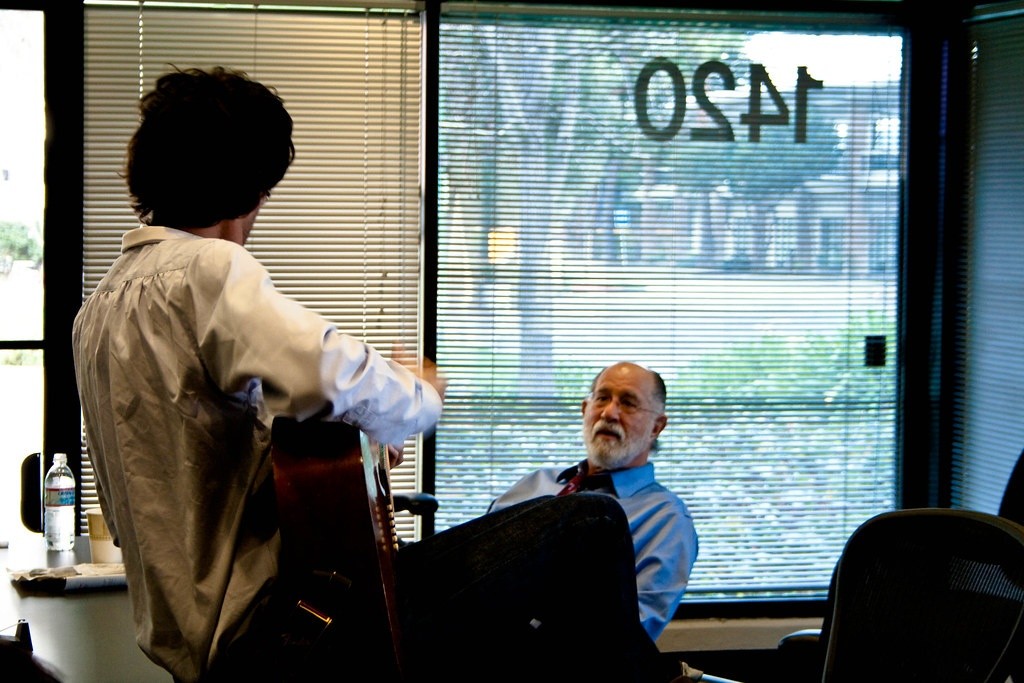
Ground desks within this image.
[0,532,178,683]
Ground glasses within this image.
[586,393,662,418]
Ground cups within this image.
[85,508,122,563]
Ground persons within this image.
[487,362,699,645]
[71,67,448,683]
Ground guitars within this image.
[270,418,403,674]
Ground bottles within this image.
[44,453,76,551]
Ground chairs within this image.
[680,508,1024,683]
